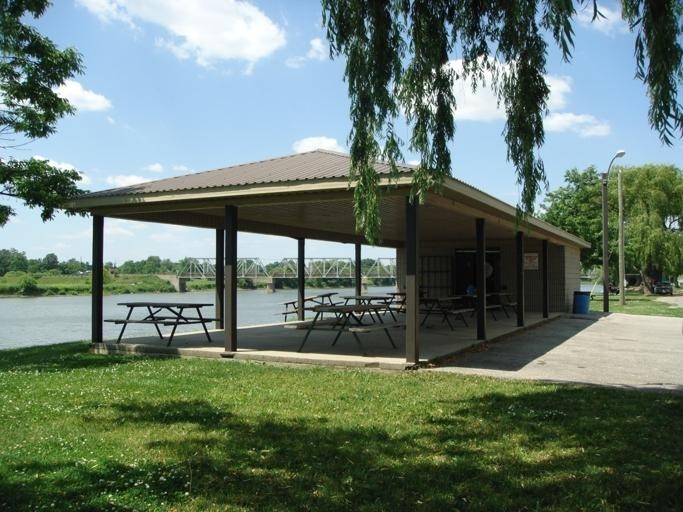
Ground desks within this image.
[114,302,214,347]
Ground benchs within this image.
[148,315,220,343]
[276,277,523,358]
[103,318,188,348]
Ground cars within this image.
[650,280,673,296]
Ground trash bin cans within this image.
[572,291,591,315]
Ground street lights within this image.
[600,149,627,313]
